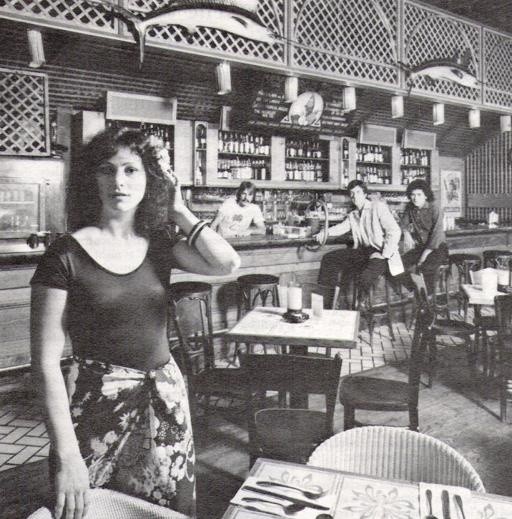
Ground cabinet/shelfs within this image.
[74,109,193,187]
[0,173,45,239]
[194,128,433,192]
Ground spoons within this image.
[241,497,305,515]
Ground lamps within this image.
[432,102,446,126]
[214,61,232,96]
[26,24,47,68]
[499,115,510,132]
[391,95,404,119]
[283,77,297,103]
[342,87,356,110]
[469,110,480,130]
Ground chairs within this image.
[248,354,343,468]
[304,426,488,494]
[169,250,512,439]
[24,489,184,518]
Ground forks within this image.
[256,481,329,499]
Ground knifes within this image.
[242,486,330,511]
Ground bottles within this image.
[356,165,390,184]
[356,144,388,162]
[218,159,267,179]
[111,122,171,148]
[401,150,428,166]
[344,169,349,187]
[200,129,205,148]
[285,160,324,182]
[344,144,348,158]
[286,140,321,158]
[401,167,427,184]
[219,132,269,154]
[286,280,302,314]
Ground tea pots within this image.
[495,256,512,294]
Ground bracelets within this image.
[186,218,211,249]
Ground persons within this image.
[29,125,240,518]
[398,179,449,308]
[317,179,405,309]
[210,180,267,236]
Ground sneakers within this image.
[358,296,369,312]
